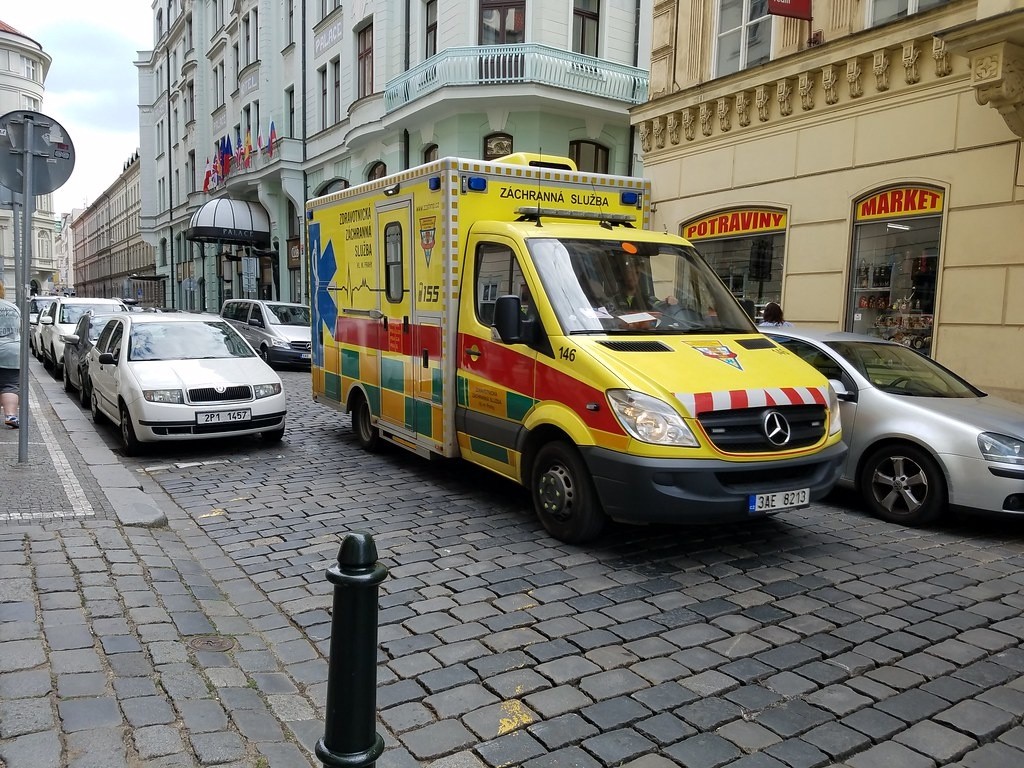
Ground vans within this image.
[219,299,311,364]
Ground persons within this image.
[0,281,21,427]
[702,283,793,329]
[857,258,933,348]
[581,265,679,314]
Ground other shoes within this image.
[5,416,19,427]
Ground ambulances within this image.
[305,152,847,540]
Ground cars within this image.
[748,327,1024,522]
[29,296,126,376]
[87,313,286,452]
[60,308,119,406]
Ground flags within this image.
[203,122,277,191]
